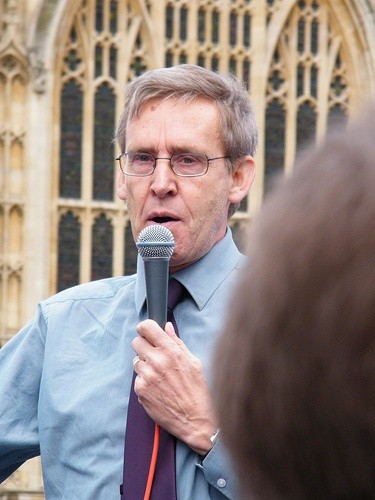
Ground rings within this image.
[133,360,140,372]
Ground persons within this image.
[213,105,374,500]
[2,64,256,499]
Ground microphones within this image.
[135,225,176,332]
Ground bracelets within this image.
[210,423,221,443]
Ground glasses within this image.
[115,152,229,176]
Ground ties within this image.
[114,302,189,500]
